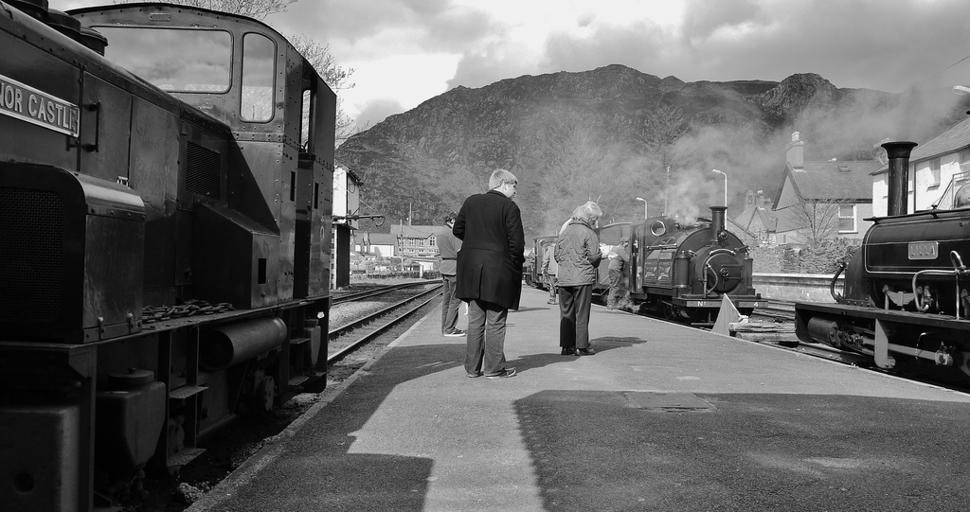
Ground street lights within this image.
[636,197,648,219]
[951,86,970,97]
[712,168,728,229]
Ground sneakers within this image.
[486,368,517,379]
[465,370,482,379]
[444,328,465,337]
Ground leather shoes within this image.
[561,346,576,355]
[575,347,596,356]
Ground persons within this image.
[541,244,558,305]
[554,200,603,356]
[452,169,526,379]
[436,212,466,337]
[607,236,630,310]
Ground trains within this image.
[0,3,342,512]
[522,204,772,328]
[792,137,968,391]
